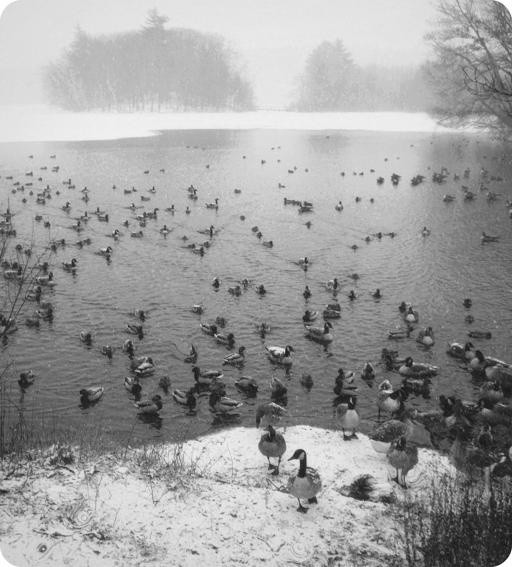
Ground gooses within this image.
[0,166,512,514]
